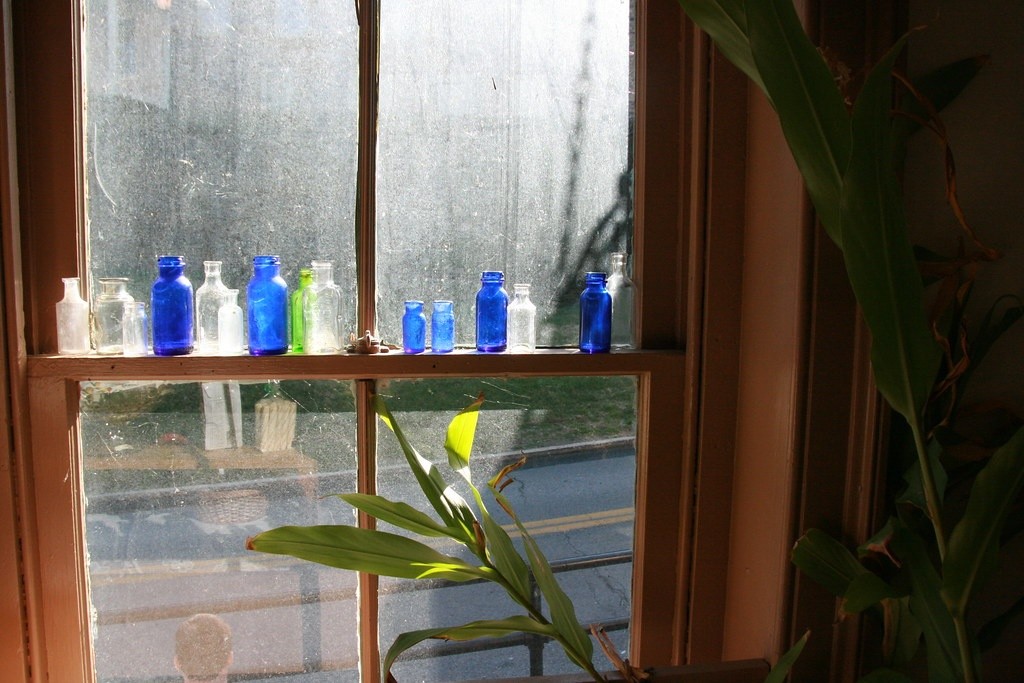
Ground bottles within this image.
[431,300,453,352]
[476,271,508,353]
[93,277,148,358]
[56,277,90,357]
[292,260,343,356]
[507,284,535,354]
[247,255,289,357]
[578,272,612,354]
[606,252,638,354]
[403,300,426,354]
[195,261,244,356]
[150,255,194,356]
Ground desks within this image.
[80,448,317,683]
